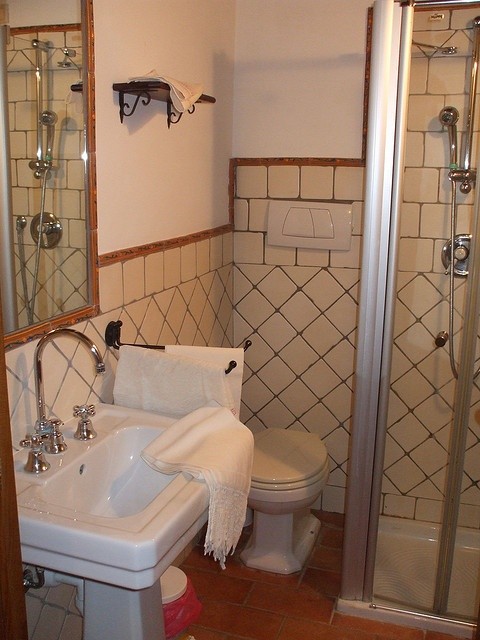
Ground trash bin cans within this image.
[160,566,202,640]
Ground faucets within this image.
[34,328,106,437]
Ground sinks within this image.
[13,406,212,589]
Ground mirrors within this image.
[2,0,103,351]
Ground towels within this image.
[112,345,241,421]
[165,345,245,422]
[128,67,205,113]
[140,398,255,570]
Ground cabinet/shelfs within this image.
[112,80,218,129]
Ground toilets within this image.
[241,428,328,575]
